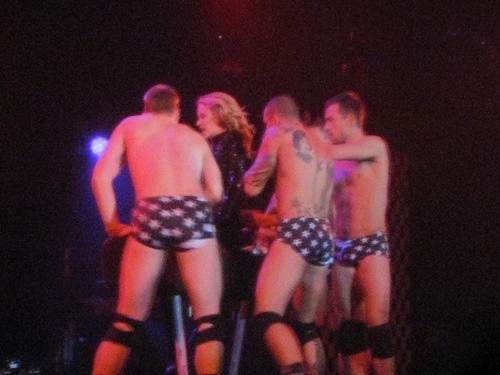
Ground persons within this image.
[166,91,258,374]
[323,92,395,374]
[241,95,340,375]
[88,85,229,375]
